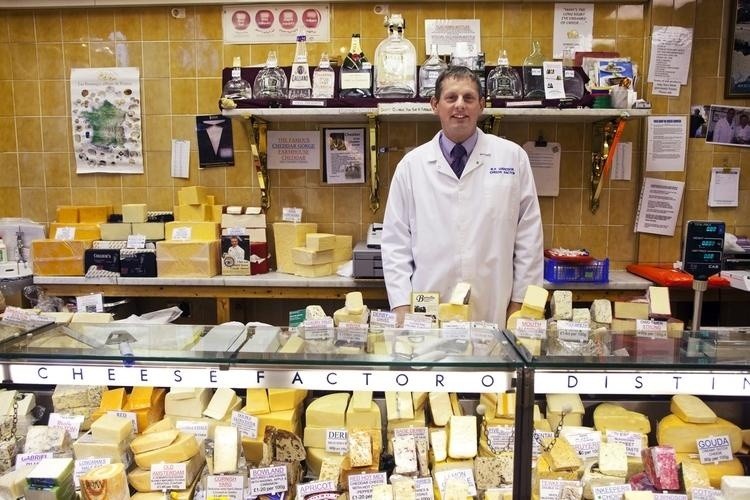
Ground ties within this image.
[450,145,467,179]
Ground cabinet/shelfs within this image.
[40,316,520,498]
[502,324,749,499]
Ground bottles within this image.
[288,26,312,98]
[419,44,449,99]
[486,48,523,100]
[559,49,585,103]
[310,51,335,99]
[373,14,418,99]
[252,49,289,97]
[338,34,373,99]
[520,39,546,99]
[224,56,253,101]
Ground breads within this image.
[277,292,504,372]
[272,222,353,278]
[0,306,113,349]
[507,286,685,357]
[531,392,750,500]
[31,185,268,278]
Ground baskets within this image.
[544,256,609,283]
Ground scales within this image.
[681,220,750,360]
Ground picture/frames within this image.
[722,1,749,102]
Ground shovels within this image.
[104,330,138,368]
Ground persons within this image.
[711,107,736,143]
[223,236,246,261]
[690,107,706,136]
[734,114,750,144]
[377,61,548,328]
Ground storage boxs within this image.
[29,187,268,282]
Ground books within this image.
[190,320,284,353]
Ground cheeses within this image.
[0,384,517,500]
